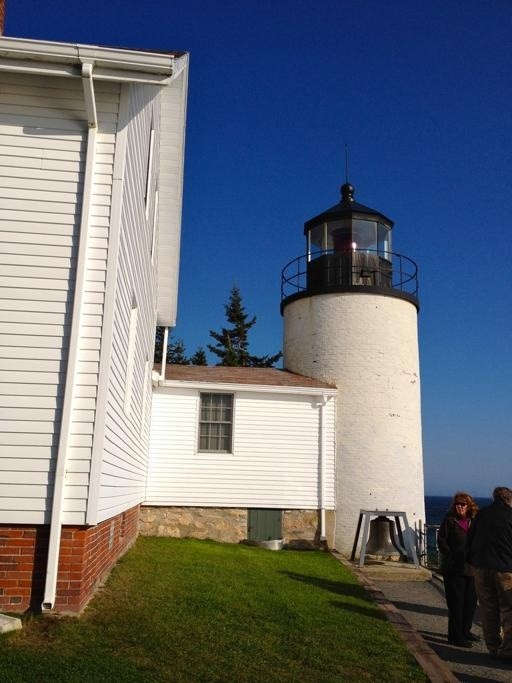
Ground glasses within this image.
[454,502,467,506]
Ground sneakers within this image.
[486,640,512,656]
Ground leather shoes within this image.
[449,632,480,647]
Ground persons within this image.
[437,491,481,647]
[465,486,512,663]
